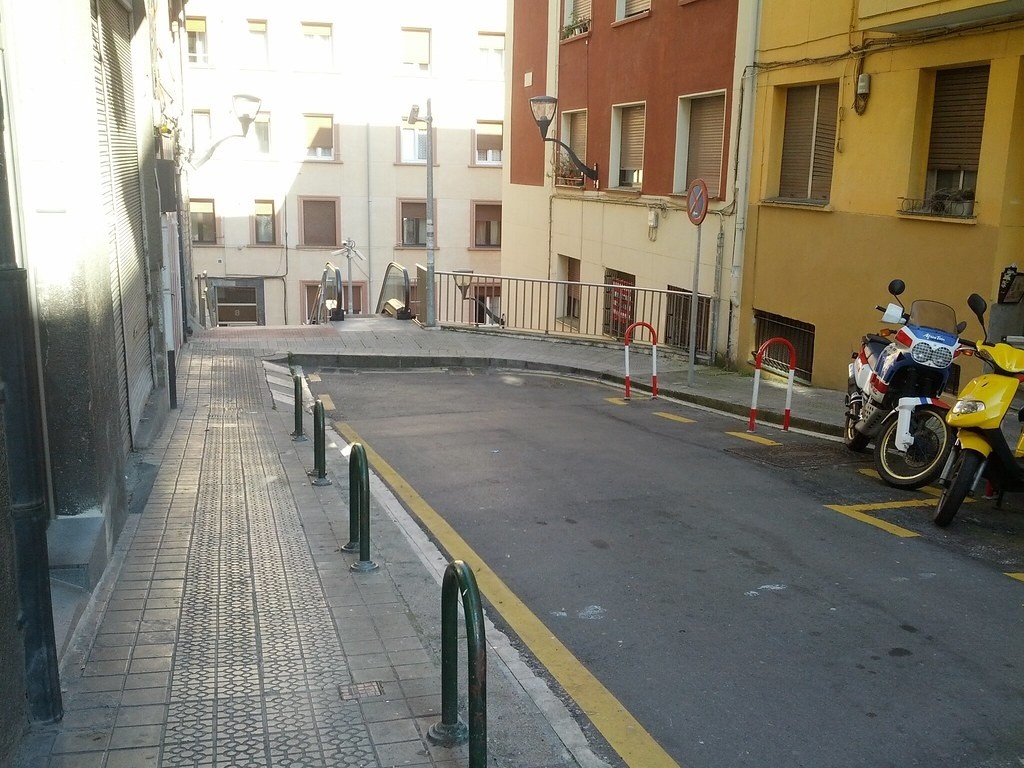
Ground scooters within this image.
[933,266,1024,529]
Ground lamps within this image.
[187,93,262,171]
[528,93,600,189]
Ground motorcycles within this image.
[841,280,979,490]
